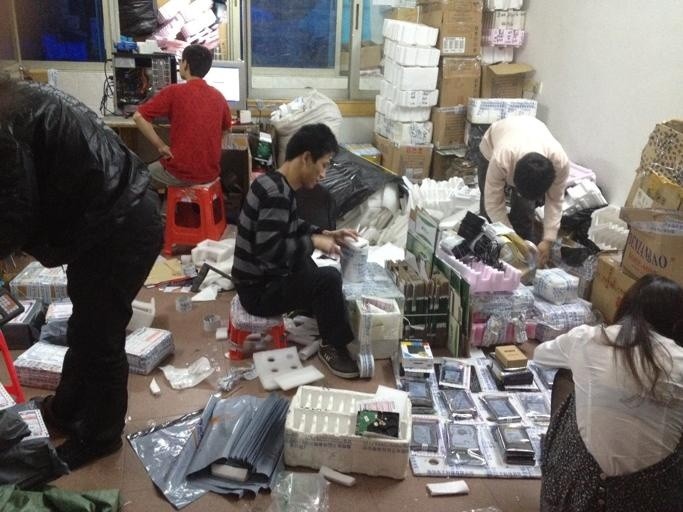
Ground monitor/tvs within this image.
[202,60,248,111]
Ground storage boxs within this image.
[345,0,538,188]
[589,119,683,324]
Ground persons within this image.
[132,44,231,233]
[474,111,570,268]
[228,122,362,381]
[519,272,682,512]
[0,63,165,464]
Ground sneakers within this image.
[315,340,361,381]
[52,422,125,470]
[29,393,65,432]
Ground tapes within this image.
[357,315,374,378]
[515,240,538,264]
[175,295,192,312]
[204,313,221,333]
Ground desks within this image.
[97,112,262,226]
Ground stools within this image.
[228,294,289,362]
[1,330,24,406]
[162,177,227,256]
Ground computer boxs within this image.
[112,50,177,116]
